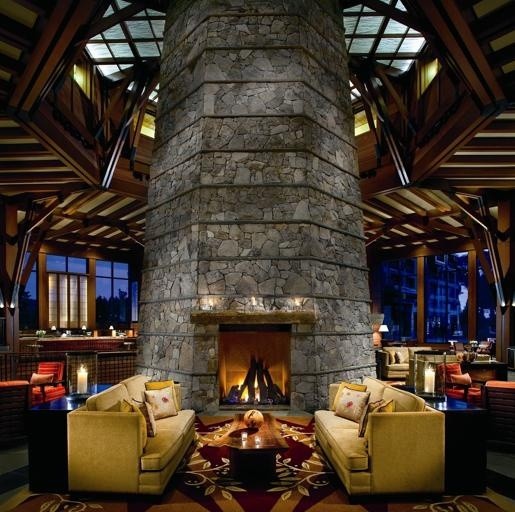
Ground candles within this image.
[251,296,256,305]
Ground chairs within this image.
[0,361,67,450]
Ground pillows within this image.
[385,348,405,364]
[116,378,181,448]
[330,381,395,438]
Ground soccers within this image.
[244,410,264,429]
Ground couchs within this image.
[65,373,197,498]
[375,344,435,377]
[314,375,447,499]
